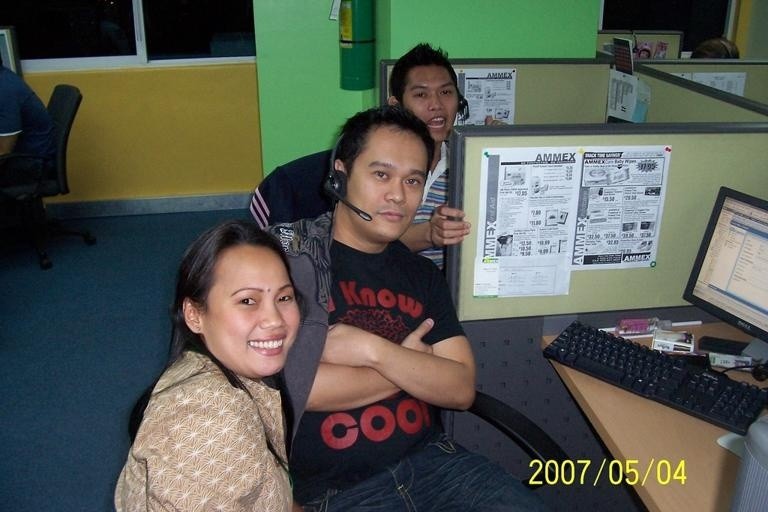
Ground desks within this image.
[537,315,767,512]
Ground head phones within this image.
[322,133,347,201]
[719,38,734,59]
[456,73,466,114]
[630,29,639,54]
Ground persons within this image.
[638,48,650,59]
[390,40,472,274]
[692,36,740,60]
[261,105,560,512]
[0,50,59,187]
[114,217,307,512]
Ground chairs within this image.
[1,82,100,272]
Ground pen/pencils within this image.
[681,352,709,357]
[621,333,652,336]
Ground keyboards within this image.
[543,320,767,435]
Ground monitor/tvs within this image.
[682,185,767,366]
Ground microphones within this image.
[321,181,373,221]
[459,99,471,121]
